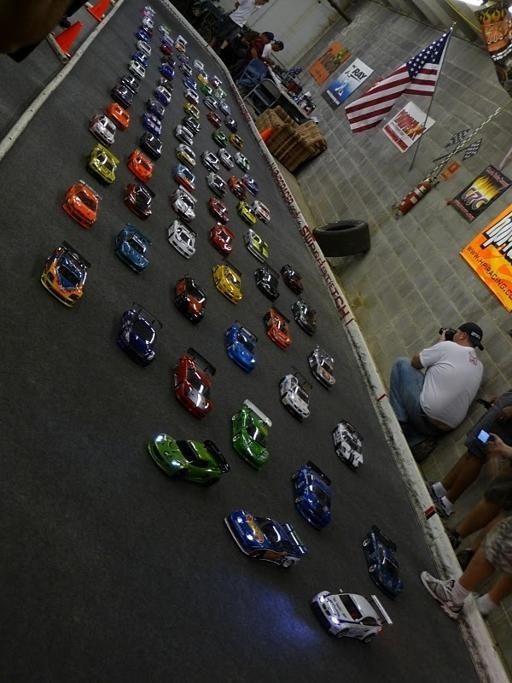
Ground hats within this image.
[459,323,484,351]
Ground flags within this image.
[342,28,450,132]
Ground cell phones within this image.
[476,427,494,446]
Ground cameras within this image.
[438,327,457,341]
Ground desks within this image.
[267,64,310,119]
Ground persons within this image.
[39,240,90,308]
[360,522,403,594]
[443,431,511,568]
[311,588,391,647]
[65,179,102,232]
[387,321,483,440]
[80,3,367,529]
[209,0,270,55]
[425,387,511,520]
[224,506,309,566]
[419,515,511,621]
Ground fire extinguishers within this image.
[397,178,432,216]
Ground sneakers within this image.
[421,571,466,619]
[427,481,473,570]
[412,438,437,462]
[473,590,489,622]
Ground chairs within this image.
[254,104,327,172]
[237,56,281,113]
